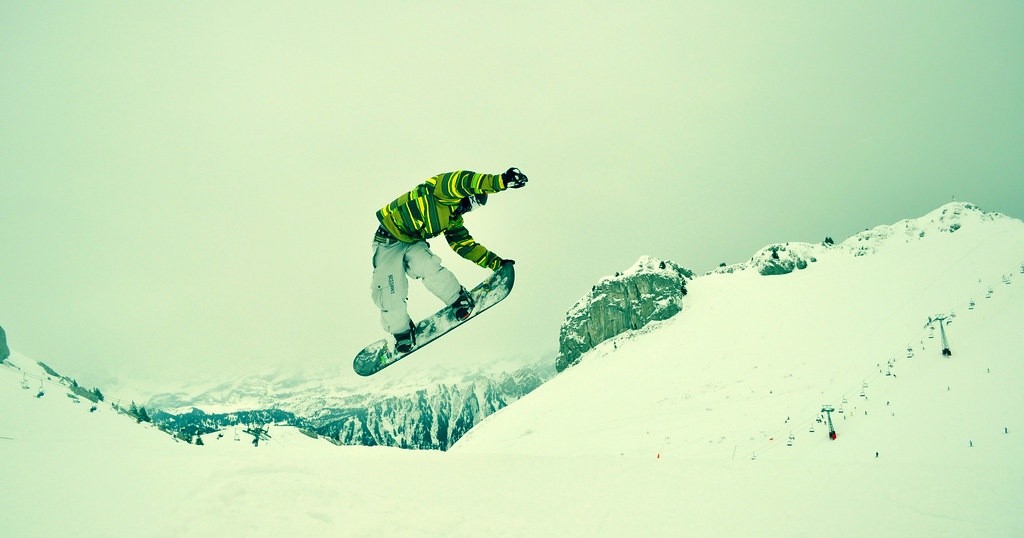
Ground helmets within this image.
[462,194,488,211]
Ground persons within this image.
[371,167,527,353]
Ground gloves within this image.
[501,260,515,267]
[502,167,528,188]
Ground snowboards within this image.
[353,262,516,377]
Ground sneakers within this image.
[452,293,475,321]
[394,320,416,353]
